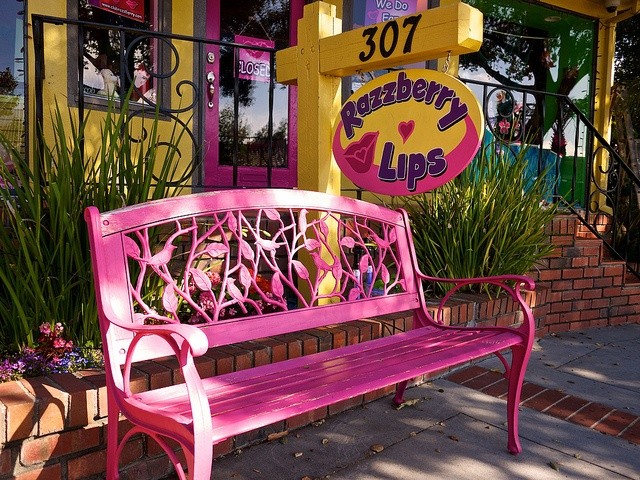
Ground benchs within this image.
[82,188,536,480]
